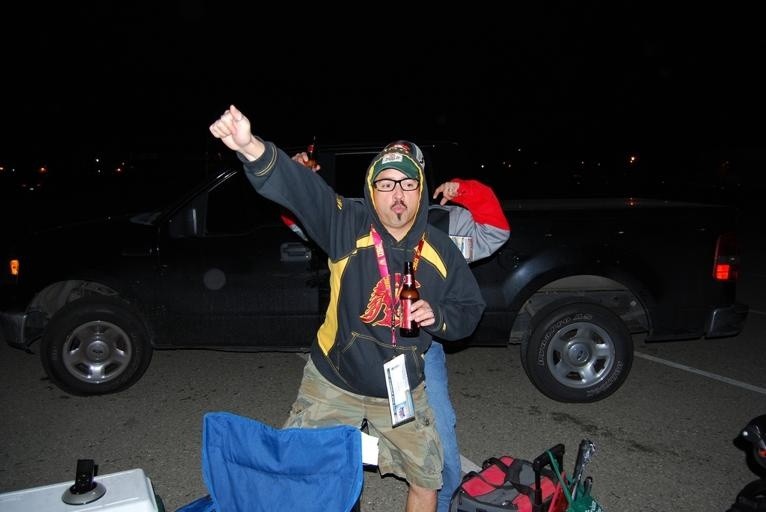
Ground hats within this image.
[371,156,419,181]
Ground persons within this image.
[395,406,406,418]
[208,104,488,510]
[278,151,512,511]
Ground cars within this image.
[5,146,756,410]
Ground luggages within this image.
[449,444,567,512]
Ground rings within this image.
[447,188,453,192]
[234,116,244,122]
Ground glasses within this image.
[372,178,421,192]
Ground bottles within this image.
[304,134,319,173]
[398,261,419,338]
